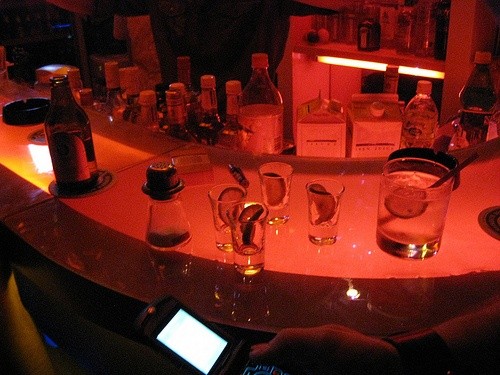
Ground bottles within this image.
[383,64,401,95]
[44,74,100,195]
[140,158,193,251]
[104,56,199,145]
[194,74,224,147]
[399,79,439,149]
[216,80,243,150]
[80,87,105,115]
[340,0,452,60]
[456,51,499,146]
[237,53,284,155]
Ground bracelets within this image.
[381,326,456,375]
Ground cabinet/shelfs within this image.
[291,0,500,159]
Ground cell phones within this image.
[136,295,302,375]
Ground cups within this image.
[208,183,247,253]
[258,162,294,226]
[377,157,455,261]
[305,178,345,247]
[226,201,269,278]
[316,14,340,42]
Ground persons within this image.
[249,299,500,375]
